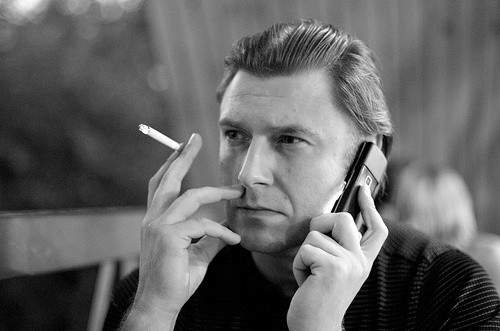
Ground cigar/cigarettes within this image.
[138,123,183,153]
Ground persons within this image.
[390,160,499,293]
[103,18,499,330]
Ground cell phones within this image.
[324,140,389,243]
